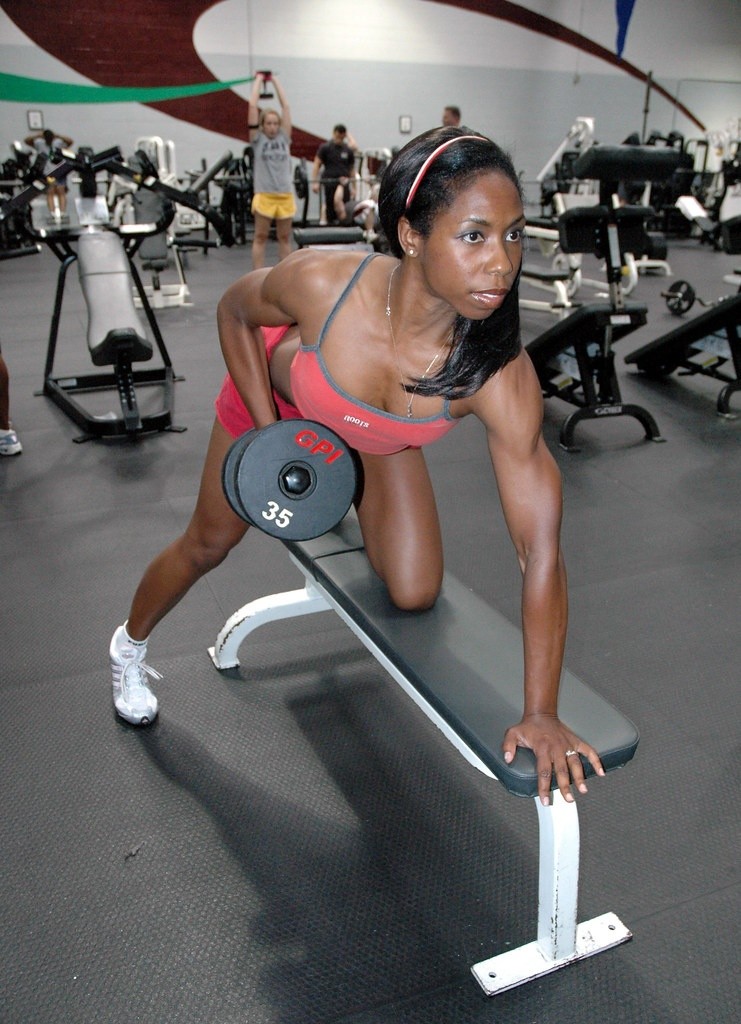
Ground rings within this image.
[566,751,580,757]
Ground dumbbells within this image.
[221,418,358,543]
[257,70,274,99]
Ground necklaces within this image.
[386,263,453,418]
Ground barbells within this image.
[660,280,722,315]
[293,166,382,198]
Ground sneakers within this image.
[0,429,23,455]
[109,625,163,725]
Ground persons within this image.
[441,106,460,126]
[248,72,298,273]
[311,124,353,227]
[352,182,389,254]
[24,129,74,221]
[0,344,24,455]
[105,125,606,805]
